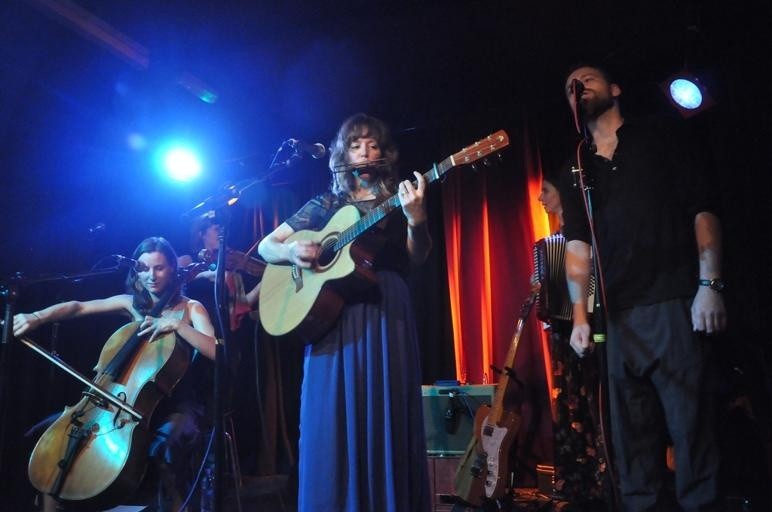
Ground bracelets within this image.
[30,312,43,323]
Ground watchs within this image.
[695,277,728,294]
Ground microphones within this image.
[571,78,587,137]
[444,392,460,434]
[288,138,326,160]
[113,254,140,269]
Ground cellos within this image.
[28,249,218,501]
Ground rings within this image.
[400,190,409,196]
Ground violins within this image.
[224,248,266,275]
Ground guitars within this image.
[259,130,510,346]
[454,293,536,507]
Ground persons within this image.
[9,235,219,512]
[175,209,265,468]
[530,168,604,512]
[254,112,440,510]
[560,60,731,512]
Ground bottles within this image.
[199,468,217,512]
